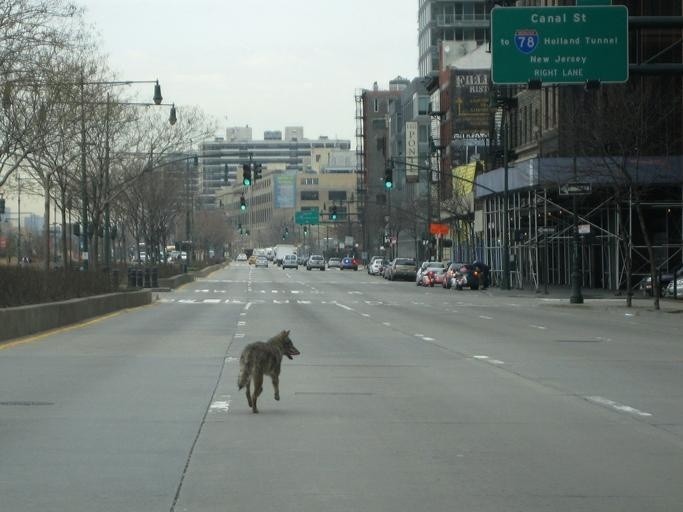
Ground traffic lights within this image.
[240,197,246,210]
[243,163,251,185]
[330,205,338,221]
[384,167,393,190]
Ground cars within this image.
[640,267,683,298]
[237,240,361,271]
[368,256,479,289]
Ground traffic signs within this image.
[295,210,320,225]
[492,6,629,83]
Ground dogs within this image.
[237,329,301,414]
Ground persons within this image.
[472,260,490,289]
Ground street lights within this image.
[105,92,177,265]
[82,81,161,269]
